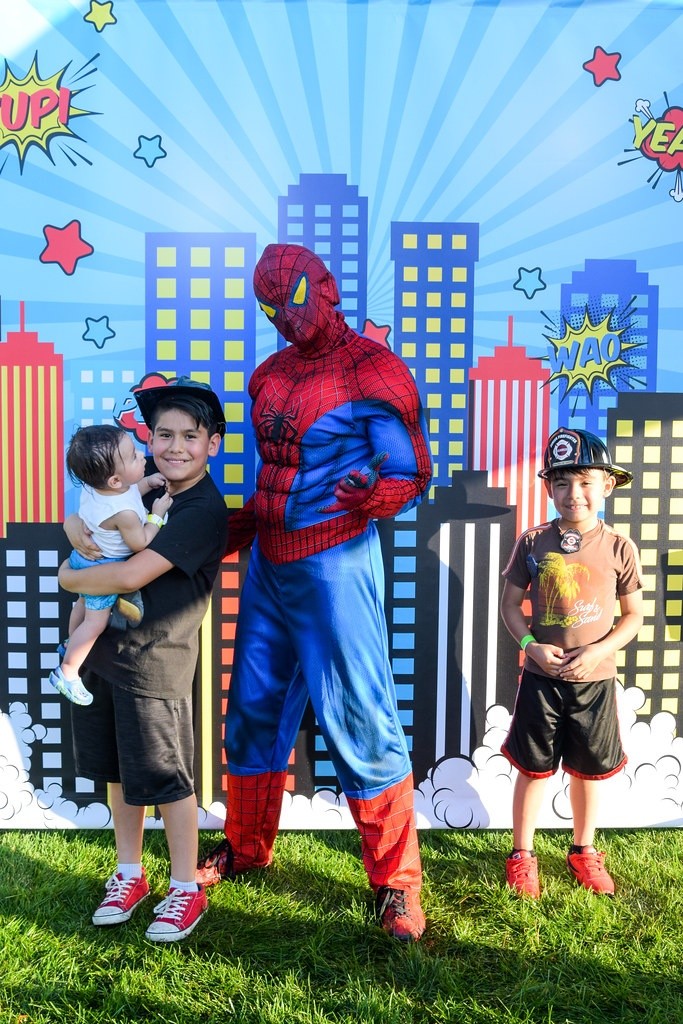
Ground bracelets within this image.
[147,514,163,528]
[520,634,536,650]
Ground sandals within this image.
[59,638,71,659]
[48,668,93,706]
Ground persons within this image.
[193,245,435,942]
[501,426,645,900]
[55,382,230,941]
[48,425,174,706]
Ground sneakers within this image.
[376,885,426,941]
[566,849,615,895]
[508,846,541,902]
[194,835,272,889]
[91,869,151,925]
[145,884,209,942]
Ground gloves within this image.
[315,452,389,513]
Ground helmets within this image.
[133,378,226,438]
[537,428,635,488]
